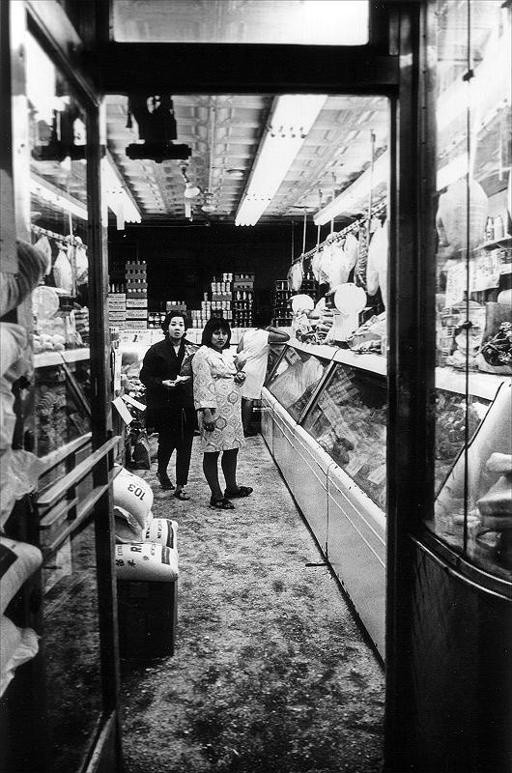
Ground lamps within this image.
[234,94,328,228]
[80,147,143,224]
[201,189,215,212]
[312,145,391,226]
[180,165,200,198]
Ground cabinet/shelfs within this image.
[31,171,124,559]
[261,337,511,662]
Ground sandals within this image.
[210,496,235,508]
[156,471,175,489]
[224,486,253,497]
[174,489,190,499]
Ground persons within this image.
[139,310,199,501]
[235,302,290,437]
[191,315,255,510]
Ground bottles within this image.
[234,272,254,327]
[272,271,322,327]
[486,205,511,240]
[167,300,186,306]
[149,311,168,327]
[108,259,148,292]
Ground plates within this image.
[332,283,367,315]
